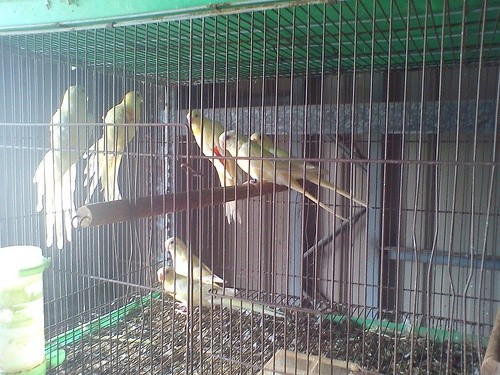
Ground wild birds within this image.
[186,111,242,224]
[248,130,373,211]
[34,85,94,251]
[83,92,144,204]
[219,129,352,222]
[164,237,227,284]
[155,266,224,316]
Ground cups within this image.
[1,246,46,375]
